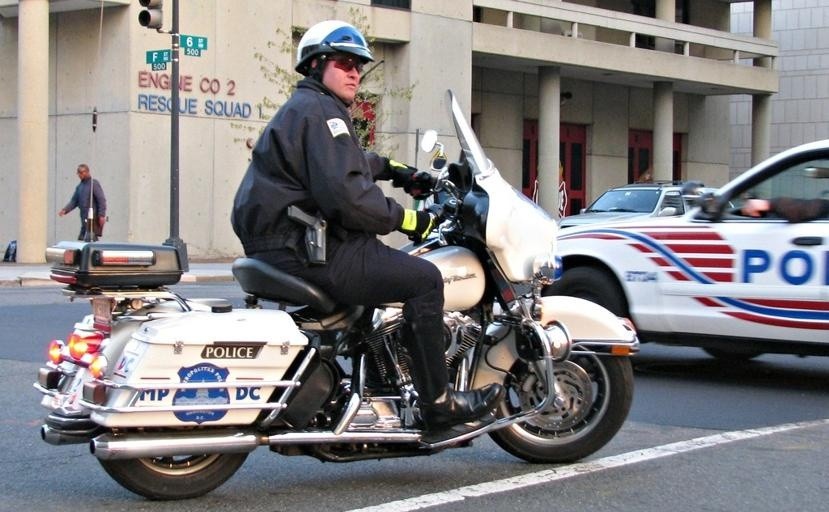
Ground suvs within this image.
[560,181,735,229]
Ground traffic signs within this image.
[180,35,208,50]
[146,48,170,62]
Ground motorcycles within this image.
[22,87,641,497]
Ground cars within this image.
[547,141,829,365]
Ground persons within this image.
[228,18,509,441]
[57,163,109,242]
[741,193,829,223]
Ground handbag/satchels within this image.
[93,215,103,236]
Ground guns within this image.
[287,205,328,268]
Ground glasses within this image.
[329,53,363,73]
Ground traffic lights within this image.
[138,0,163,29]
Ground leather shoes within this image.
[421,382,506,430]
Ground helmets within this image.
[295,20,375,76]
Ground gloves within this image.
[400,209,435,244]
[389,159,430,200]
